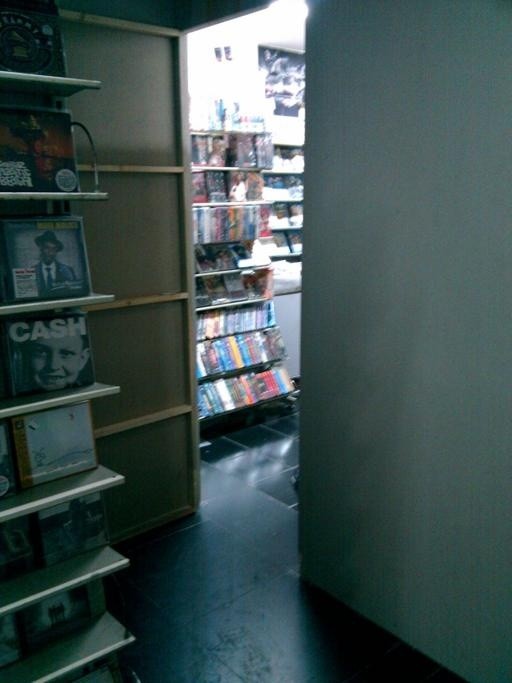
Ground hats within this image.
[34,230,65,253]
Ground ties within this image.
[44,266,54,289]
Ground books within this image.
[1,0,112,666]
[190,120,304,419]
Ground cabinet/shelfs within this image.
[0,5,304,683]
